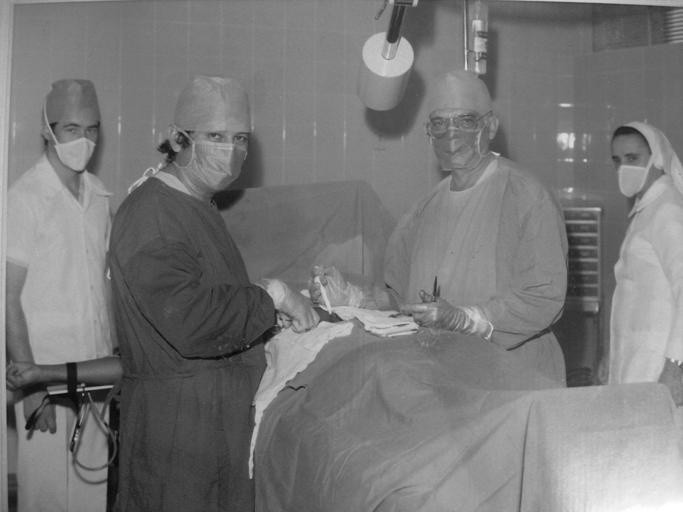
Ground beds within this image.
[212,181,683,512]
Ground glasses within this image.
[426,111,494,130]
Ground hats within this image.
[173,76,251,132]
[425,70,493,120]
[43,79,102,124]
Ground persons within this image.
[108,74,322,511]
[306,68,571,389]
[592,117,683,420]
[5,355,122,391]
[5,77,118,511]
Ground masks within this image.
[618,155,654,198]
[49,131,97,172]
[181,129,246,196]
[431,122,489,170]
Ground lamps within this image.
[356,0,419,112]
